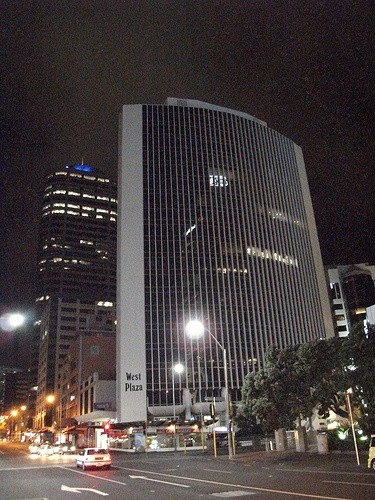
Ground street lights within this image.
[46,395,62,445]
[185,319,234,460]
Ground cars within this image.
[76,448,112,471]
[367,434,375,472]
[29,441,76,457]
